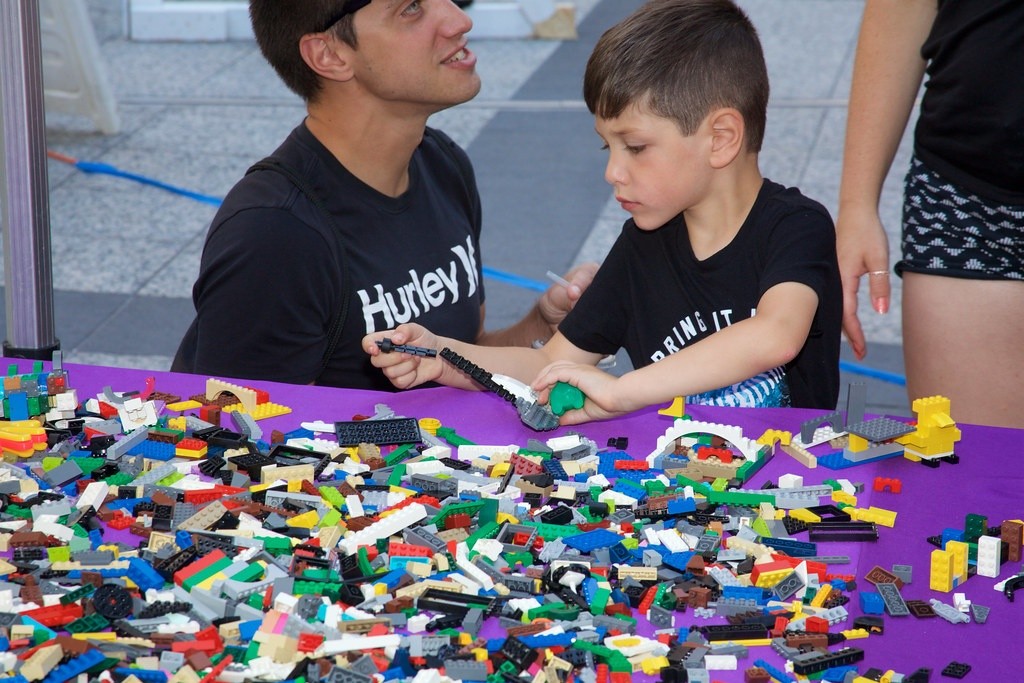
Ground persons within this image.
[834,0,1024,429]
[170,0,601,393]
[362,0,844,426]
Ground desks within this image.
[0,352,1024,683]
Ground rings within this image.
[869,271,890,275]
